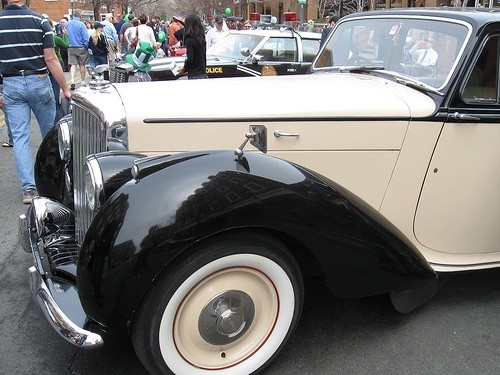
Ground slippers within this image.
[2,141,13,147]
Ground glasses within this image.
[245,26,250,28]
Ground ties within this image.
[420,49,427,63]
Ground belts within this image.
[0,67,48,77]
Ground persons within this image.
[126,41,156,82]
[205,14,251,48]
[49,21,69,106]
[408,38,439,66]
[147,16,185,56]
[118,15,166,58]
[0,74,14,147]
[306,19,315,31]
[57,12,121,71]
[65,9,90,90]
[179,14,208,79]
[88,21,109,82]
[0,0,71,204]
[320,15,359,66]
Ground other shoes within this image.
[92,72,102,79]
[81,80,88,87]
[22,188,38,204]
[70,82,75,90]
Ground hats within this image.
[215,16,222,23]
[425,37,434,44]
[172,16,185,25]
[223,15,227,18]
[307,19,314,24]
[94,22,106,29]
[126,41,157,72]
[325,16,330,19]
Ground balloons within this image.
[158,30,166,43]
[124,14,131,22]
[225,8,232,16]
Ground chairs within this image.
[413,51,438,78]
